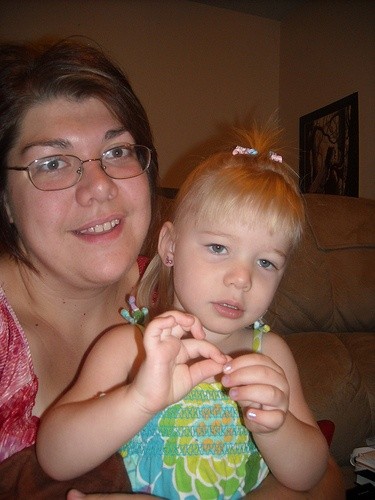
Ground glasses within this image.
[4,142,154,192]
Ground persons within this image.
[34,106,332,500]
[0,37,347,500]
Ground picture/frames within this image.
[297,91,361,201]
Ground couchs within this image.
[129,194,374,500]
